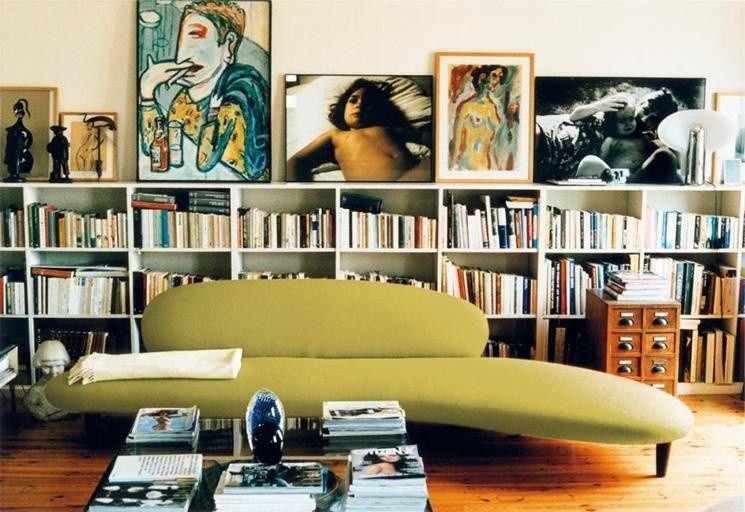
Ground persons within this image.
[75,127,99,172]
[22,340,81,422]
[287,77,432,183]
[535,87,684,183]
[450,64,518,171]
[600,102,677,183]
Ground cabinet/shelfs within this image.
[584,284,682,397]
[1,177,744,402]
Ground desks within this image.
[83,452,433,511]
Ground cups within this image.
[167,120,185,169]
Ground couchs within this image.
[46,272,692,483]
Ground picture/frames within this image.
[429,50,540,187]
[55,110,120,183]
[0,84,62,183]
[708,87,744,187]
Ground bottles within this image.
[48,124,72,182]
[148,117,168,172]
